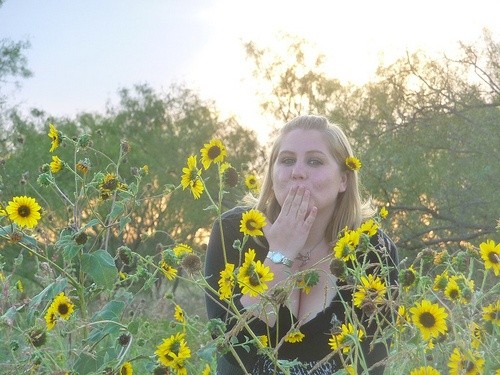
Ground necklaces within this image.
[295,235,327,265]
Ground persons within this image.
[205,116,400,375]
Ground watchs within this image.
[266,251,294,267]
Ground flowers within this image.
[343,156,362,174]
